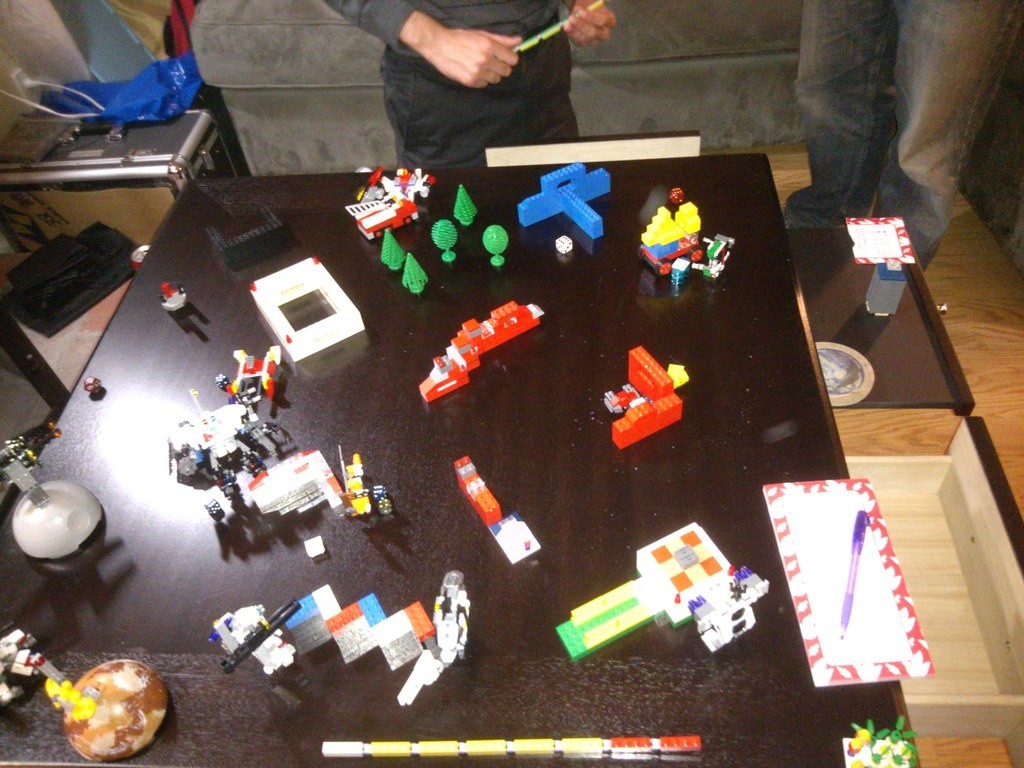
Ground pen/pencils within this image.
[840,510,870,641]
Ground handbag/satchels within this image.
[38,49,203,121]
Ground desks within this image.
[0,131,922,768]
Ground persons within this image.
[325,0,617,172]
[782,0,1024,273]
